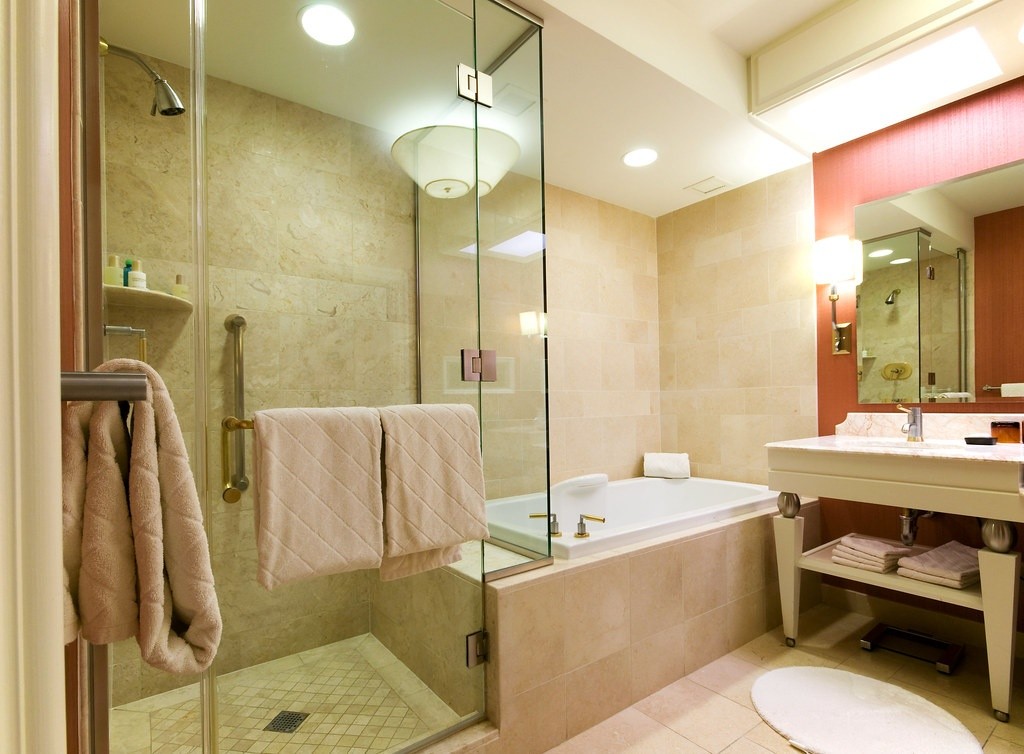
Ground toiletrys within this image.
[862,346,869,358]
[171,273,190,301]
[128,257,148,289]
[122,257,136,286]
[104,254,126,287]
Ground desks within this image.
[763,410,1024,723]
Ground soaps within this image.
[962,435,1000,447]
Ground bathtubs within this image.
[369,473,821,752]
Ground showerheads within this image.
[152,78,187,118]
[883,291,899,305]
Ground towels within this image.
[241,404,385,590]
[62,357,223,677]
[896,567,981,589]
[1000,382,1024,397]
[375,400,491,583]
[897,538,990,581]
[830,535,912,575]
[642,452,692,479]
[939,391,974,405]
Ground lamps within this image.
[811,233,868,355]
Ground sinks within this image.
[763,430,1024,491]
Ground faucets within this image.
[897,404,926,442]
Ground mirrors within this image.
[850,158,1024,405]
